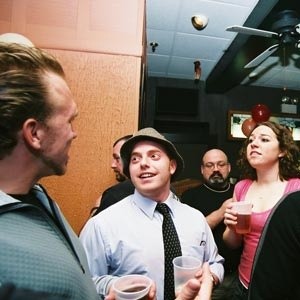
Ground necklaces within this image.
[202,182,231,193]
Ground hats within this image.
[120,128,184,179]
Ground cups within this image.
[112,274,153,300]
[230,201,252,234]
[172,256,202,299]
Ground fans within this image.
[226,10,300,78]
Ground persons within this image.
[78,127,226,300]
[222,121,300,300]
[180,149,235,258]
[88,134,135,217]
[0,31,105,300]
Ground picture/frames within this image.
[227,111,300,143]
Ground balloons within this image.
[251,102,271,121]
[242,118,257,138]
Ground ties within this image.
[155,203,182,300]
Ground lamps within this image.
[193,61,202,83]
[191,16,209,31]
[278,32,296,91]
[281,96,299,114]
